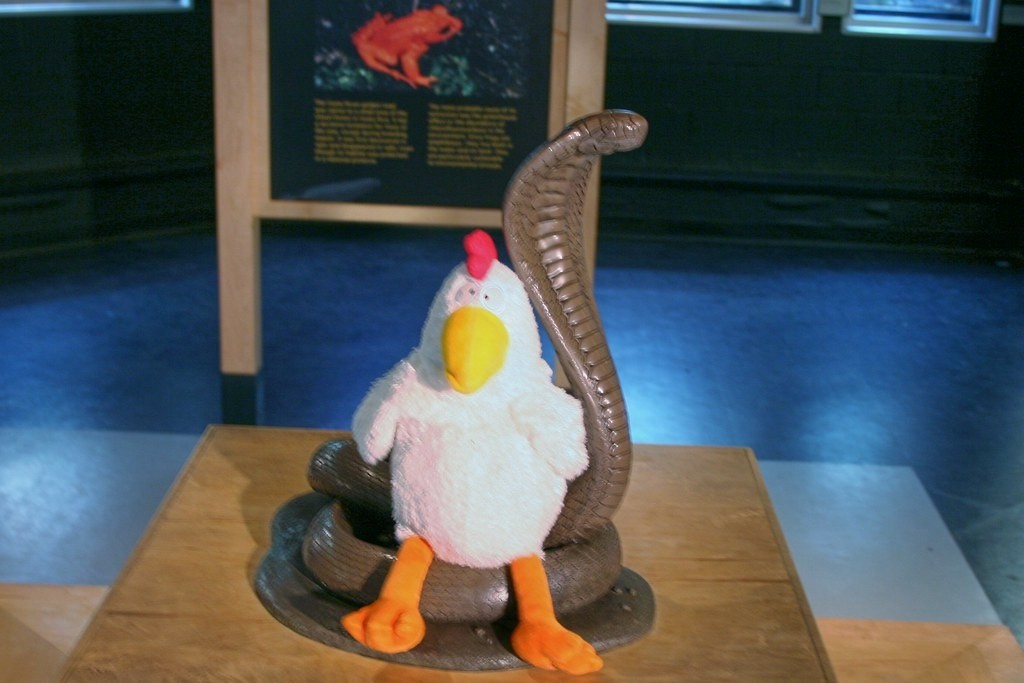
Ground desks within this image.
[57,424,834,683]
